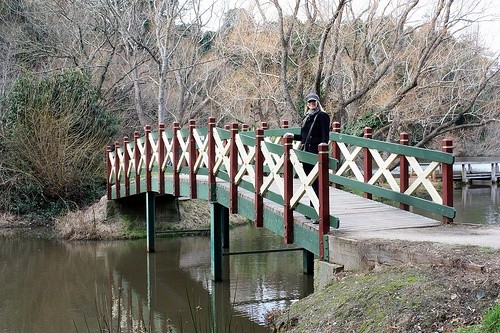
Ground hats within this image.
[305,93,320,102]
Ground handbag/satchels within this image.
[294,142,306,179]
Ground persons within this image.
[284,93,330,223]
